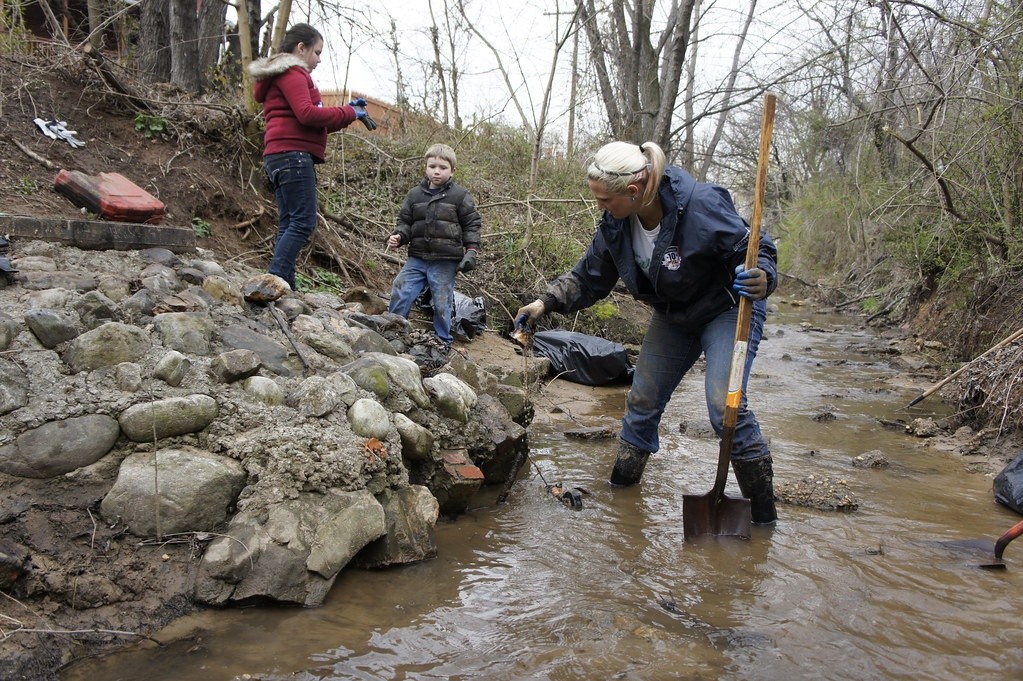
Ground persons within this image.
[513,141,777,524]
[387,143,484,352]
[247,24,370,292]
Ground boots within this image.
[609,438,651,488]
[730,454,777,525]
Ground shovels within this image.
[681,92,779,547]
[912,519,1023,568]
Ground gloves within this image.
[514,298,546,334]
[733,264,767,300]
[350,98,377,131]
[455,250,476,274]
